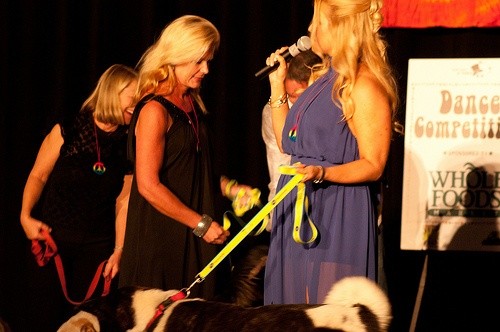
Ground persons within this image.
[21,64,138,332]
[264,0,398,305]
[262,51,322,231]
[122,16,256,292]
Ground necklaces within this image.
[289,72,336,142]
[92,117,106,174]
[176,94,201,152]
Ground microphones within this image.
[255,36,312,79]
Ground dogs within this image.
[55,309,102,332]
[125,270,392,332]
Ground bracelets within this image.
[313,166,326,183]
[269,92,289,108]
[225,179,236,197]
[193,214,213,237]
